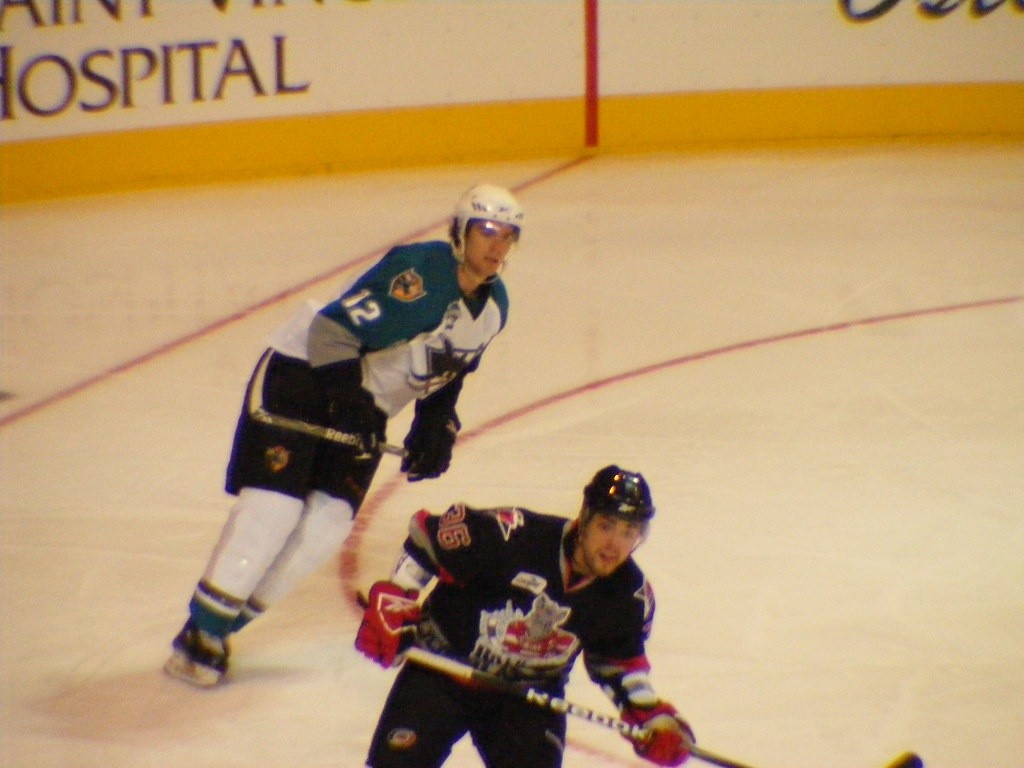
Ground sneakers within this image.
[163,616,231,686]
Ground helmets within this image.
[449,184,523,255]
[584,465,656,521]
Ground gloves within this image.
[320,384,377,462]
[354,580,420,669]
[401,412,461,483]
[619,701,695,768]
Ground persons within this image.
[171,182,524,674]
[355,465,696,768]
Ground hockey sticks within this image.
[251,345,412,460]
[354,588,926,767]
